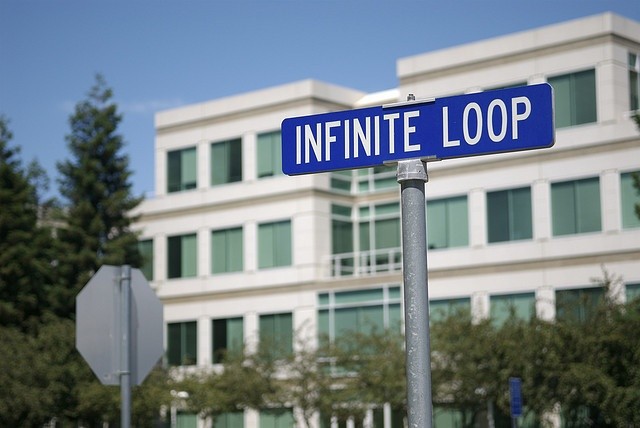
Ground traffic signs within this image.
[280,80,557,176]
